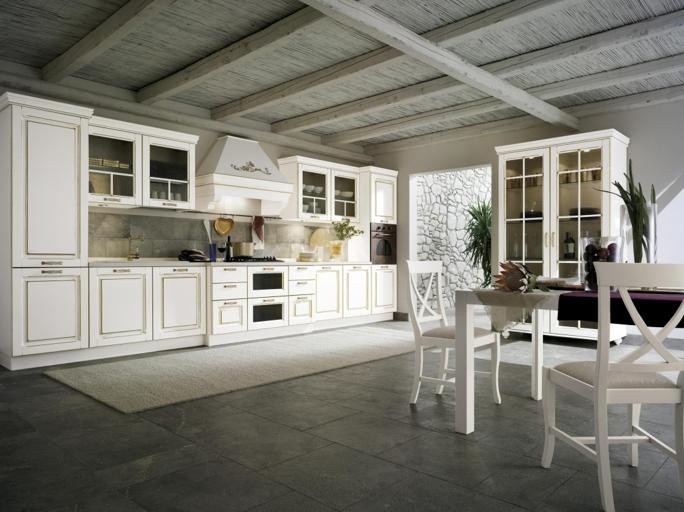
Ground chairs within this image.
[541,258,682,510]
[405,257,504,414]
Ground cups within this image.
[171,192,182,200]
[151,191,166,199]
[209,243,217,262]
[580,235,623,293]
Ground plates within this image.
[89,158,129,169]
[559,167,600,184]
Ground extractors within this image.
[195,134,293,218]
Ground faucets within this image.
[127,233,145,261]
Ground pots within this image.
[231,241,256,257]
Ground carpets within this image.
[34,325,444,416]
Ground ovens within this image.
[370,222,396,264]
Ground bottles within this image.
[562,232,576,259]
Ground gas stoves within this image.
[223,256,285,262]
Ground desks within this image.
[450,275,683,440]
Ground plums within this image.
[584,243,616,290]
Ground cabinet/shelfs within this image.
[356,165,399,227]
[0,264,208,372]
[210,262,250,348]
[1,88,95,270]
[289,262,316,335]
[493,127,629,348]
[319,263,398,335]
[89,114,200,219]
[277,155,359,225]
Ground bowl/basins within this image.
[303,201,323,213]
[303,184,324,193]
[335,189,353,200]
